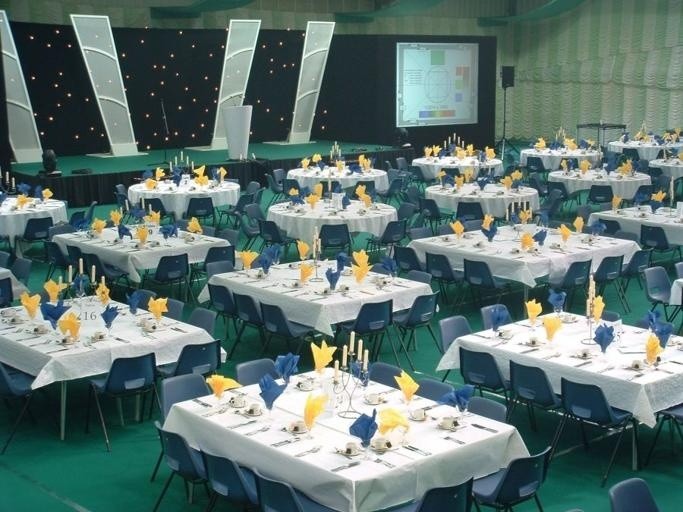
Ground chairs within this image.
[1,132,682,510]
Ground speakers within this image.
[503,66,514,88]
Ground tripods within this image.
[493,93,520,159]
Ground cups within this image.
[439,224,621,259]
[471,309,683,381]
[5,298,189,348]
[229,263,408,295]
[191,375,501,473]
